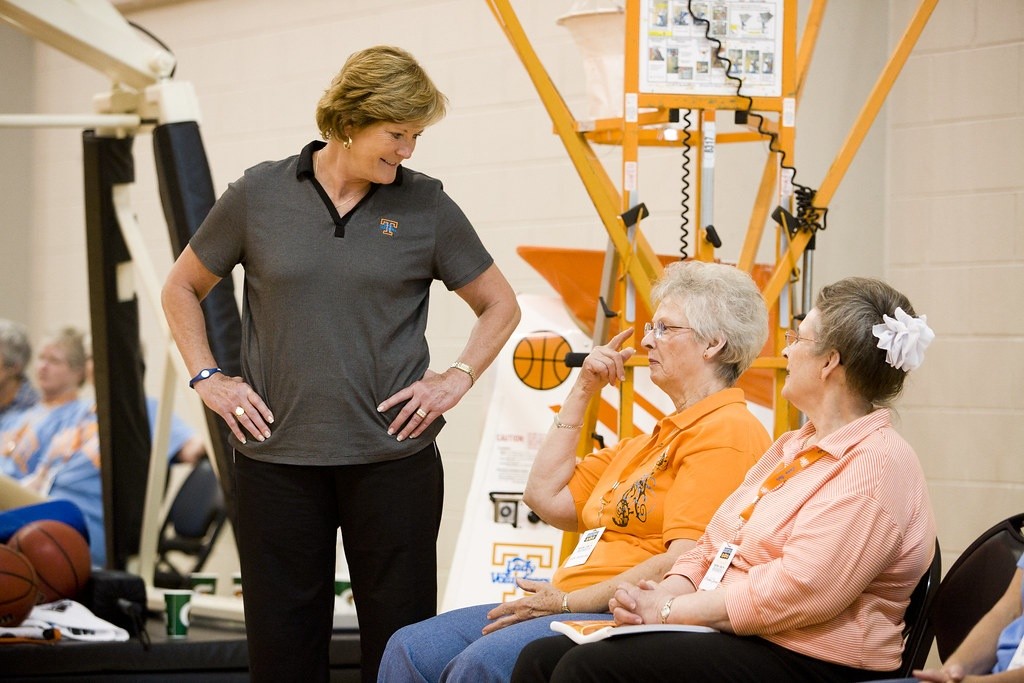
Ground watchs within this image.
[190,368,222,389]
[562,593,570,613]
[450,362,476,388]
[661,597,674,624]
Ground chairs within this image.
[904,513,1024,677]
[151,457,228,585]
[897,538,941,676]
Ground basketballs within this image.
[6,519,91,605]
[0,543,39,627]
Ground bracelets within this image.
[554,414,584,429]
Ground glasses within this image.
[785,329,844,369]
[643,320,696,340]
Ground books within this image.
[550,620,721,645]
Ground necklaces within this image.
[316,150,361,207]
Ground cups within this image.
[334,580,353,605]
[190,573,217,594]
[232,573,243,598]
[164,592,191,638]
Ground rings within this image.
[235,407,244,418]
[416,408,427,418]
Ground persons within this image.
[162,44,522,683]
[376,258,773,683]
[0,328,86,482]
[511,278,937,683]
[912,552,1024,683]
[1,318,38,427]
[0,340,208,572]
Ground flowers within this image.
[872,306,936,371]
